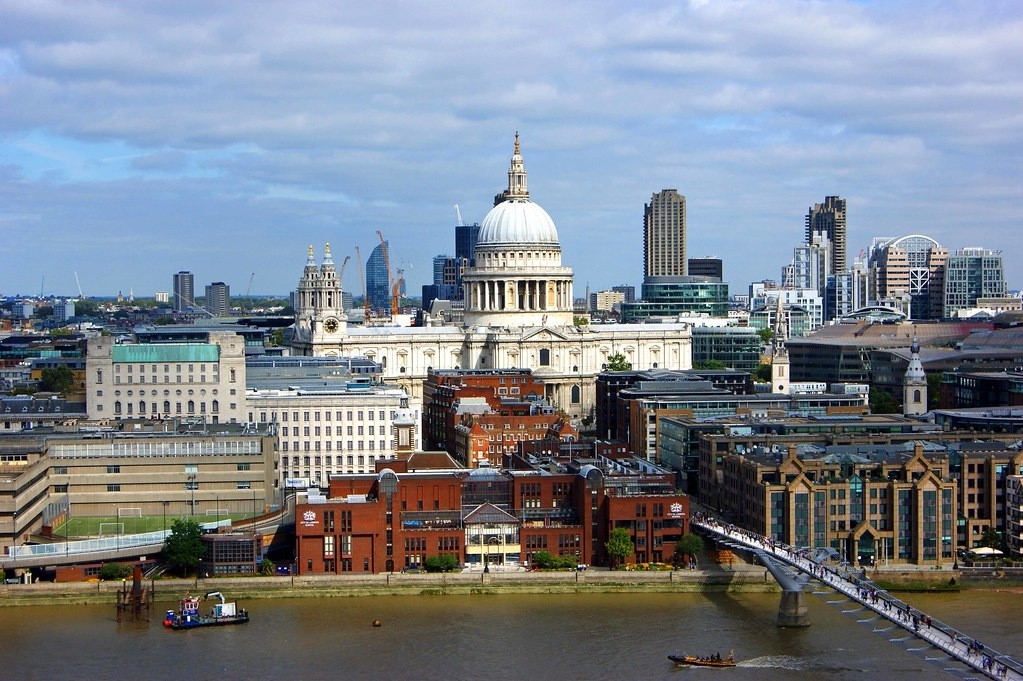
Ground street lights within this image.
[63,508,69,556]
[210,492,219,528]
[111,503,120,552]
[12,511,17,562]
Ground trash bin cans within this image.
[921,614,926,622]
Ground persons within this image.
[846,575,931,631]
[689,510,776,555]
[808,562,843,582]
[950,631,985,657]
[980,655,1008,677]
[777,542,806,565]
[695,652,720,663]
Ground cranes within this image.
[354,245,371,318]
[339,255,351,280]
[376,228,407,314]
[246,272,255,295]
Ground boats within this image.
[667,654,737,667]
[163,590,250,629]
